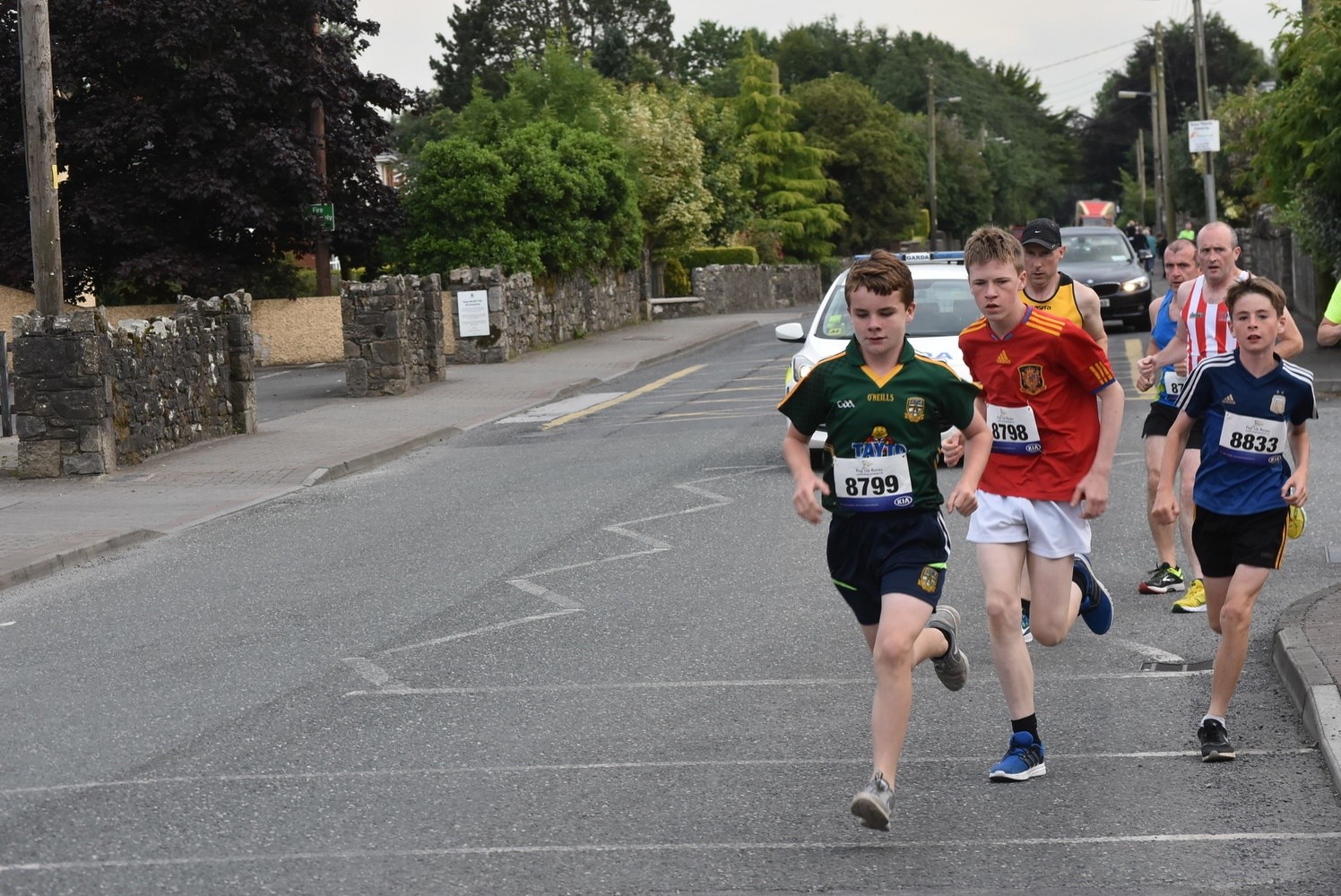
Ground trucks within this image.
[1076,199,1117,227]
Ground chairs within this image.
[955,302,984,332]
[915,302,940,335]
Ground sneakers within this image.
[929,605,970,692]
[1289,506,1307,539]
[1021,615,1034,642]
[1172,579,1208,612]
[1197,718,1236,760]
[1139,561,1185,593]
[1074,553,1114,635]
[851,771,895,830]
[988,731,1046,780]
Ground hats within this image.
[1021,218,1062,252]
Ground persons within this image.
[1136,239,1204,594]
[1138,221,1307,612]
[1019,219,1109,642]
[940,228,1125,780]
[1115,200,1195,278]
[1153,271,1318,763]
[777,250,992,832]
[1317,279,1341,346]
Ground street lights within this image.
[1117,89,1171,245]
[924,74,961,251]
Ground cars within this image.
[775,252,984,472]
[1053,225,1153,330]
